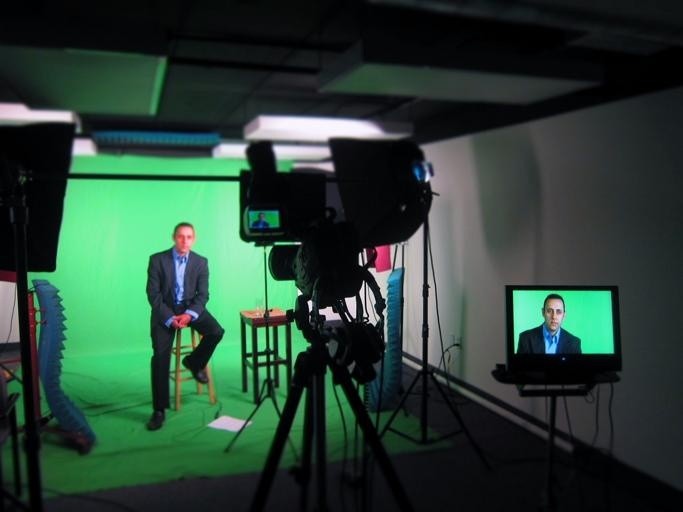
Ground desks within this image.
[240,308,291,405]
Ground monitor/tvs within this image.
[504,284,622,377]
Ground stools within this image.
[169,324,216,410]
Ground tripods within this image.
[248,320,410,512]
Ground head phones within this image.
[295,266,387,367]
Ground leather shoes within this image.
[182,354,209,384]
[147,410,164,430]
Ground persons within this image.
[515,294,582,357]
[250,210,269,231]
[143,221,223,433]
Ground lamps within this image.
[212,115,388,160]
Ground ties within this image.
[548,337,557,354]
[177,258,185,303]
